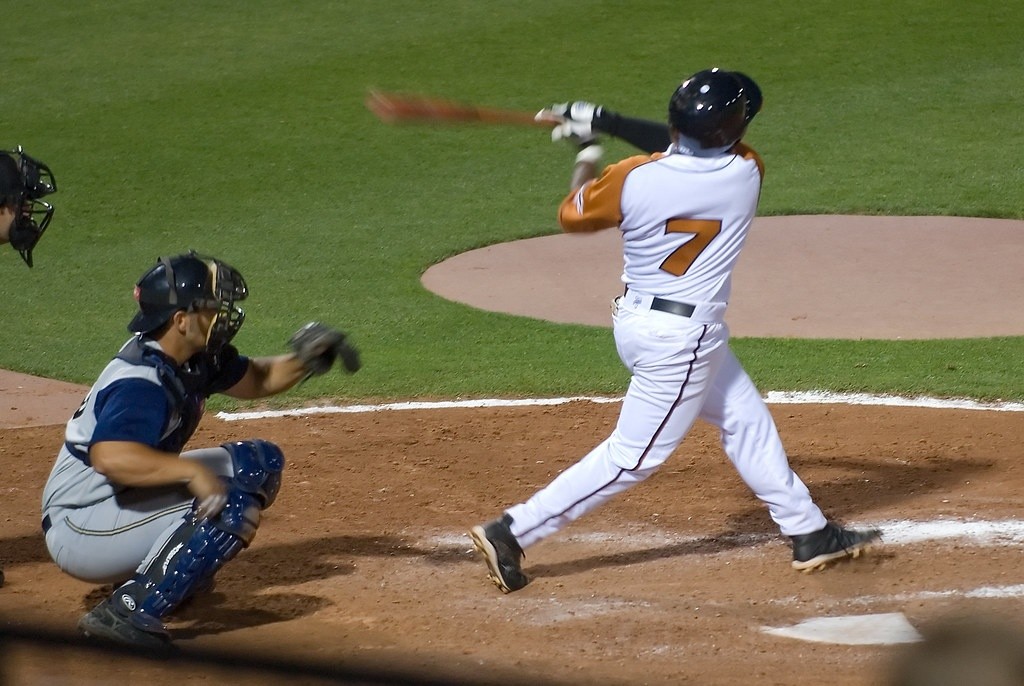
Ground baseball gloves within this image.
[287,321,365,387]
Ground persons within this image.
[469,69,884,595]
[40,251,361,652]
[0,147,56,268]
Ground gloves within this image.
[552,122,591,141]
[534,101,604,122]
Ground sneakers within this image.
[77,594,172,655]
[468,514,529,594]
[788,523,884,574]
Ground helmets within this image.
[0,150,22,202]
[128,255,216,334]
[668,68,764,153]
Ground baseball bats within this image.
[374,93,560,131]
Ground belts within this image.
[624,283,728,317]
